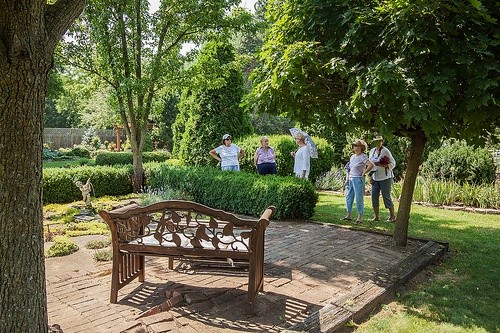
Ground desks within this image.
[169,219,250,268]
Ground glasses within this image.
[354,144,359,147]
[225,139,231,141]
[295,138,297,140]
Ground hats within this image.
[374,135,383,140]
[353,140,367,146]
[222,134,232,140]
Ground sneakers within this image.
[368,216,380,222]
[384,216,396,223]
[354,218,363,225]
[340,216,352,221]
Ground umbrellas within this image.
[289,128,319,159]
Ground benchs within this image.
[100,200,276,318]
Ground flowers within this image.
[380,156,390,176]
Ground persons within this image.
[368,136,396,222]
[209,134,244,171]
[291,133,310,179]
[340,139,374,224]
[254,136,277,176]
